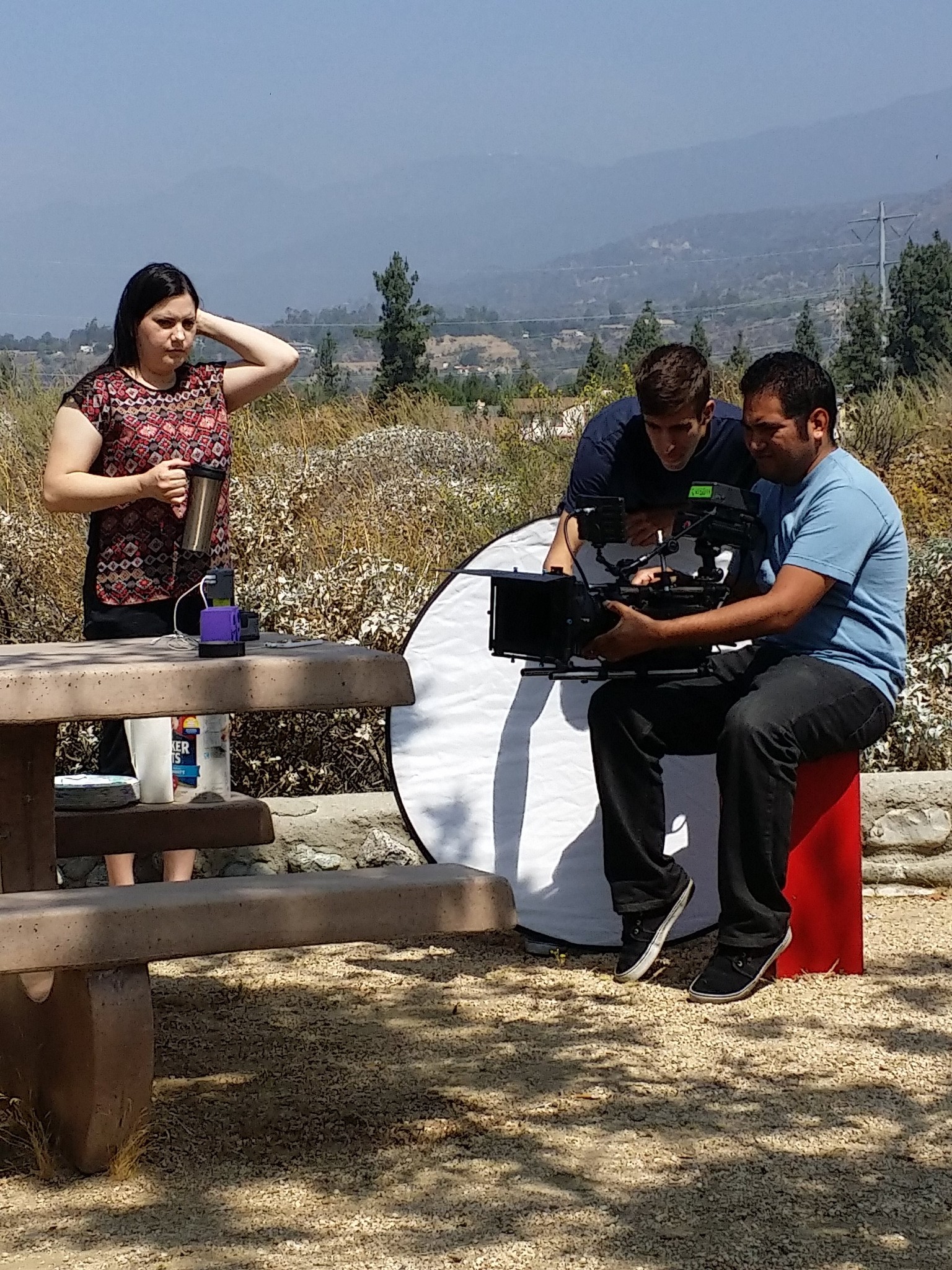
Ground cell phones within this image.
[264,639,323,648]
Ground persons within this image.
[543,344,766,578]
[42,261,299,889]
[579,352,909,1002]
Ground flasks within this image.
[178,464,227,552]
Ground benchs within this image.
[0,793,519,1179]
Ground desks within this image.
[0,632,415,1122]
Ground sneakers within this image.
[689,925,792,1003]
[615,877,695,981]
